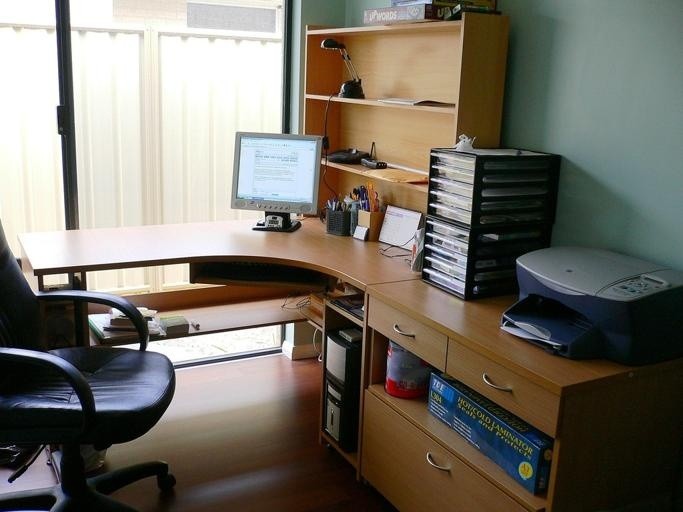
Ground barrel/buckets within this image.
[385,339,432,399]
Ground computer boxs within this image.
[325,334,362,452]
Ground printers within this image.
[500,246,683,366]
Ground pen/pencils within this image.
[326,192,346,212]
[350,185,379,212]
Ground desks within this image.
[18,216,422,484]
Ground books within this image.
[339,328,363,343]
[88,307,189,344]
[377,98,455,106]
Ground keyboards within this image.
[198,262,318,283]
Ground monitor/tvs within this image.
[230,132,323,231]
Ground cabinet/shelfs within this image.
[300,9,511,242]
[358,297,682,511]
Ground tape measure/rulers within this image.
[367,181,375,211]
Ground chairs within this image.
[0,212,180,512]
